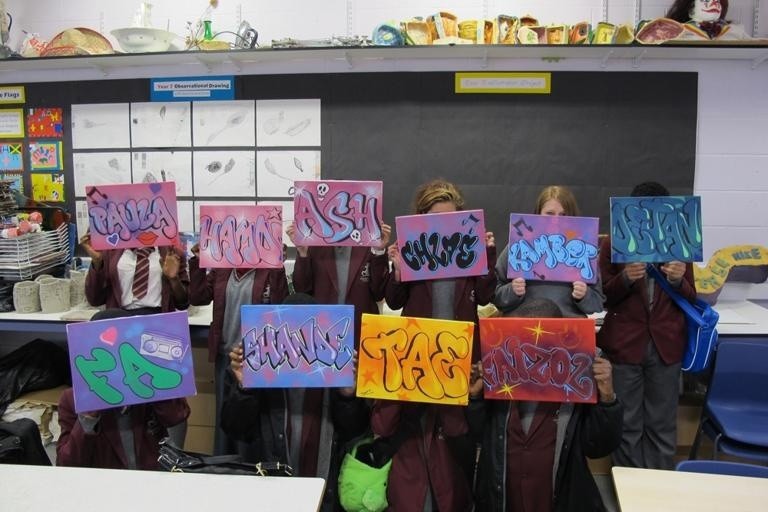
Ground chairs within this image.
[674,335,768,480]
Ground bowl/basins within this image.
[109,27,179,54]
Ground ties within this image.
[129,247,155,300]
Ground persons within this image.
[489,186,606,318]
[367,398,476,511]
[79,232,191,320]
[437,298,624,512]
[285,218,391,511]
[384,177,497,363]
[596,181,697,469]
[187,236,290,464]
[58,307,192,473]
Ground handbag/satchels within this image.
[682,297,720,373]
[158,437,294,476]
[338,438,392,512]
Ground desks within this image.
[2,264,220,349]
[2,463,326,512]
[611,466,768,512]
